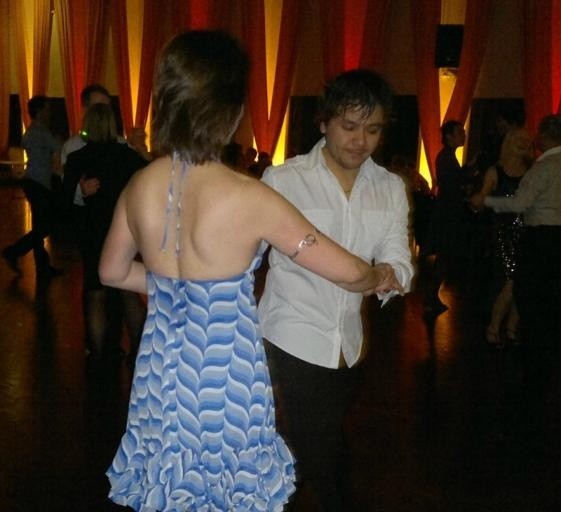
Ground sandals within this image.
[486,323,524,350]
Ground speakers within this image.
[435,25,464,67]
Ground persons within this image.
[256,70,417,510]
[97,27,406,511]
